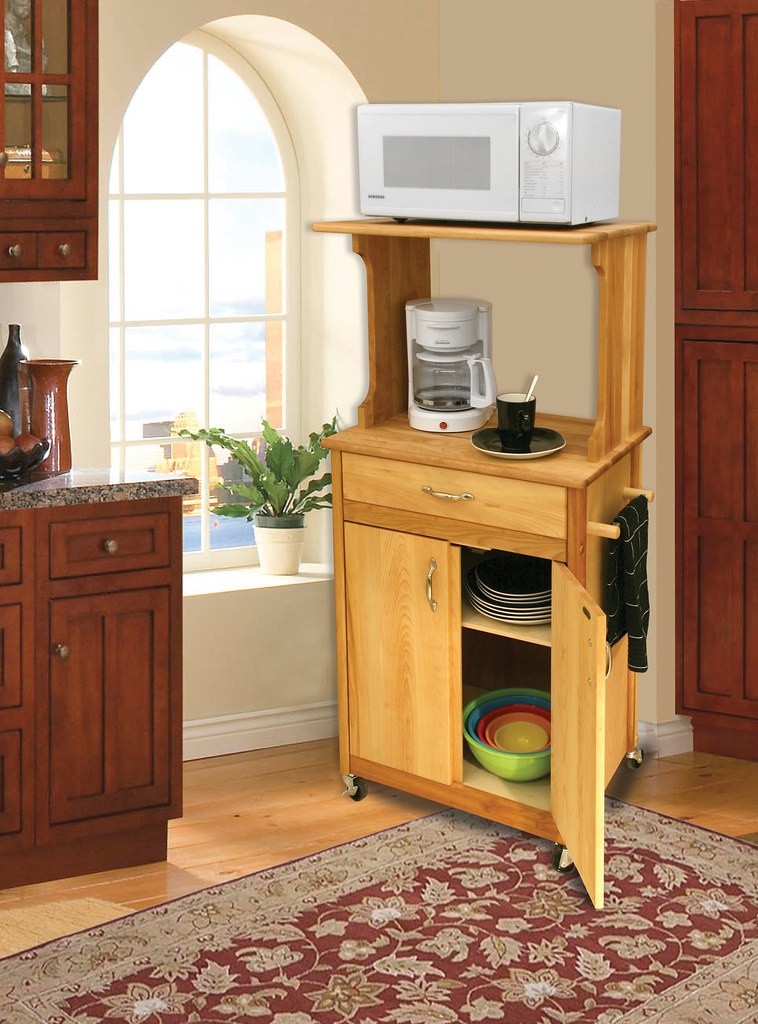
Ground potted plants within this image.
[169,416,342,527]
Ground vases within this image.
[252,527,303,575]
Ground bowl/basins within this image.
[479,704,550,744]
[469,696,551,749]
[0,438,50,481]
[485,713,550,750]
[464,688,551,781]
[493,722,548,752]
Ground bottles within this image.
[0,324,27,436]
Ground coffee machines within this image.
[405,297,498,433]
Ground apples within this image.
[0,410,42,455]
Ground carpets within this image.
[0,795,758,1024]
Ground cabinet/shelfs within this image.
[0,469,198,890]
[311,218,657,911]
[0,0,100,283]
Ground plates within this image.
[470,427,566,458]
[464,560,551,625]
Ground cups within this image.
[496,393,536,452]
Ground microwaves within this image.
[357,103,622,226]
